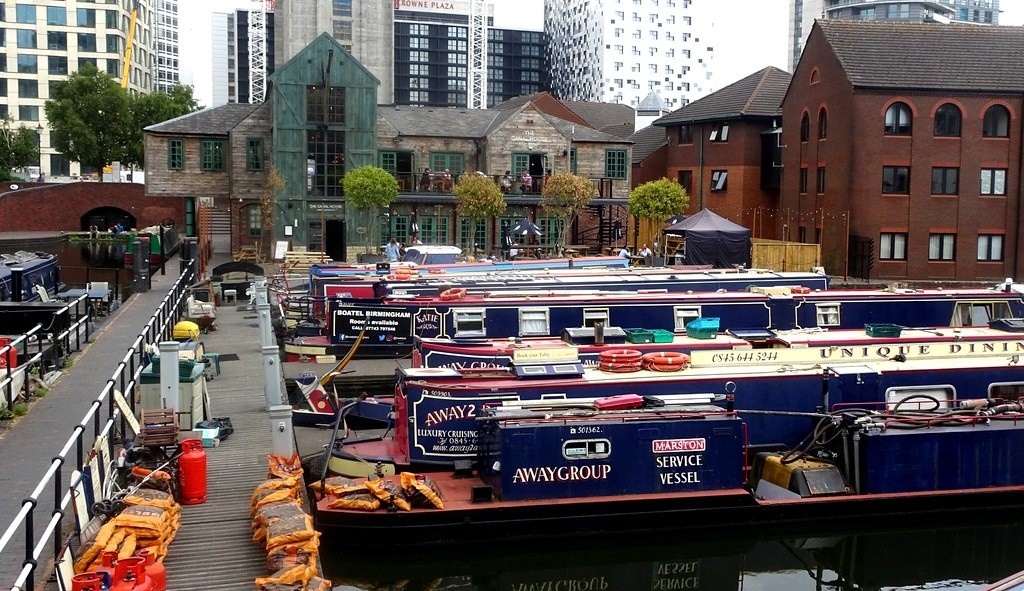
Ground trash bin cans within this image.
[153,341,205,363]
[140,353,205,431]
[189,279,211,302]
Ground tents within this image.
[662,208,751,274]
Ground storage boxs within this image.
[646,329,674,343]
[192,427,220,448]
[687,318,720,329]
[315,355,336,364]
[685,326,718,339]
[864,323,902,337]
[152,354,197,377]
[622,328,653,343]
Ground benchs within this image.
[231,245,334,273]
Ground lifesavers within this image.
[395,268,418,275]
[429,268,444,274]
[439,287,467,301]
[596,349,644,373]
[791,286,811,295]
[387,273,411,280]
[640,350,689,373]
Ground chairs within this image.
[36,284,64,303]
[396,174,542,194]
[67,296,88,322]
[246,283,256,305]
[187,295,216,326]
[87,282,108,317]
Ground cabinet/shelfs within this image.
[139,361,204,429]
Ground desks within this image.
[464,242,648,267]
[66,288,112,321]
[133,428,203,462]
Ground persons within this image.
[486,250,497,260]
[385,237,401,262]
[109,223,123,239]
[421,167,457,192]
[412,236,424,245]
[459,170,517,195]
[519,170,552,195]
[639,244,652,265]
[509,238,539,260]
[89,224,98,239]
[618,245,630,258]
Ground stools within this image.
[224,290,236,304]
[202,353,220,376]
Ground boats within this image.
[304,343,1023,552]
[0,251,69,355]
[280,244,1024,476]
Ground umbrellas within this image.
[664,213,687,225]
[507,217,543,245]
